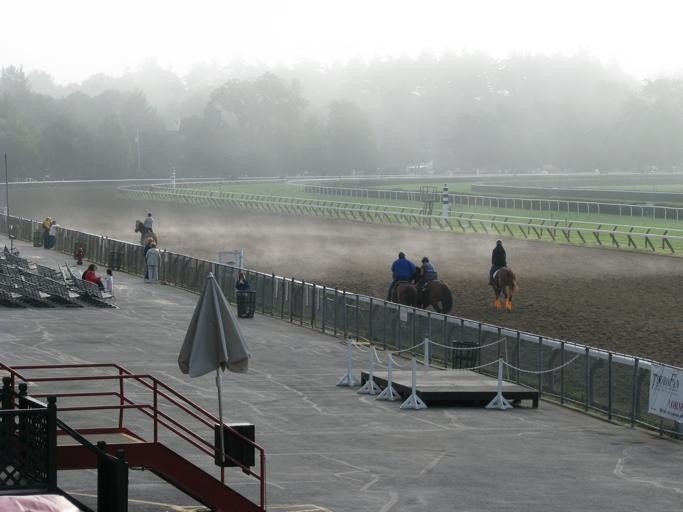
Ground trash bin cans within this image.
[236,290,257,318]
[74,242,87,260]
[452,340,479,371]
[33,231,43,247]
[108,252,121,271]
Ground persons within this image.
[417,257,437,287]
[46,219,62,249]
[42,215,50,249]
[145,242,161,283]
[236,272,250,290]
[140,212,154,243]
[387,253,418,300]
[82,263,113,295]
[142,238,155,279]
[487,237,508,285]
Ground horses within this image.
[412,265,453,314]
[391,281,420,307]
[134,219,158,247]
[491,266,518,313]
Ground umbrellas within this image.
[175,272,252,463]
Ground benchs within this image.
[0,245,118,310]
